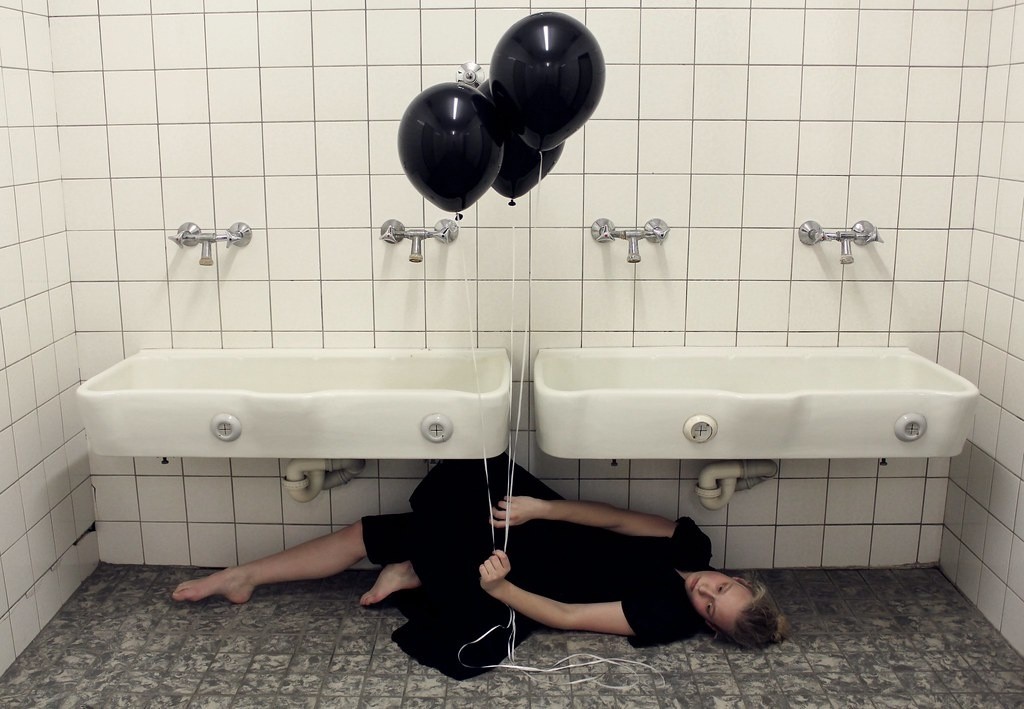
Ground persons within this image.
[172,452,788,679]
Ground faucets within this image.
[192,233,219,267]
[406,231,424,263]
[623,229,642,263]
[837,230,855,265]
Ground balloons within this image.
[397,82,505,222]
[475,11,606,207]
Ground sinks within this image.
[76,348,510,459]
[532,347,981,460]
